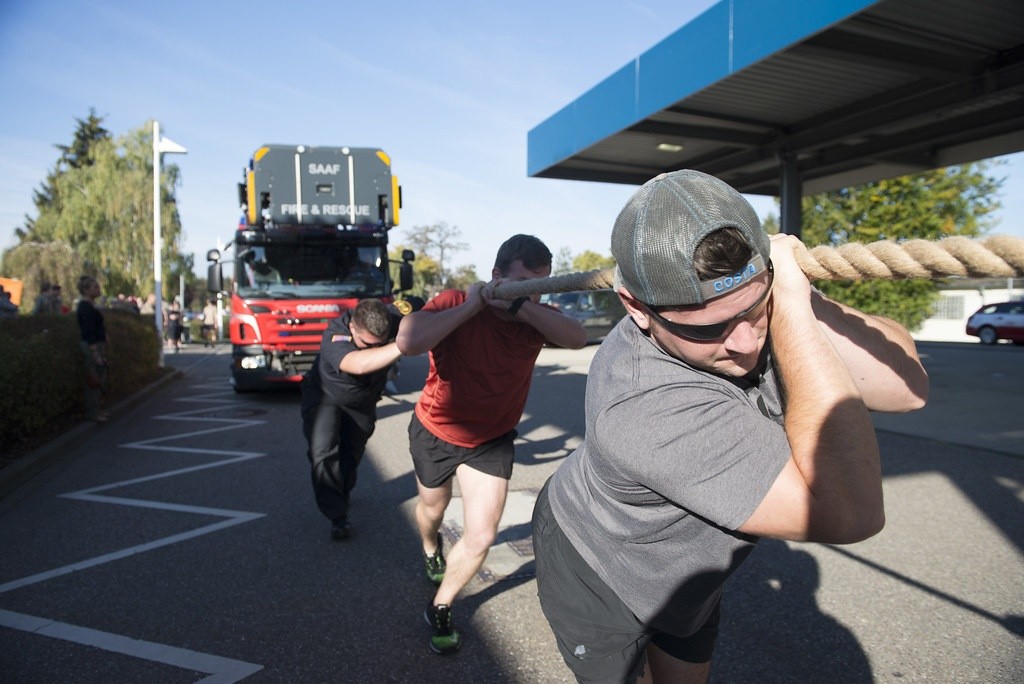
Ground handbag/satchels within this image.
[85,358,113,398]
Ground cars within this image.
[545,290,628,349]
[965,302,1024,348]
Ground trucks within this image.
[205,143,417,393]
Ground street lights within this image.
[149,118,189,367]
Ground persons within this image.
[301,294,426,542]
[532,170,930,684]
[75,275,112,423]
[396,234,589,653]
[203,298,217,348]
[0,284,183,354]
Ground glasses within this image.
[618,257,774,340]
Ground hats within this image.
[610,169,771,305]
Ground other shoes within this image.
[97,409,110,417]
[385,379,399,395]
[85,414,107,421]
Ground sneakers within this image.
[421,532,446,584]
[344,491,349,499]
[424,597,462,653]
[330,515,348,538]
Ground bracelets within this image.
[507,295,530,316]
